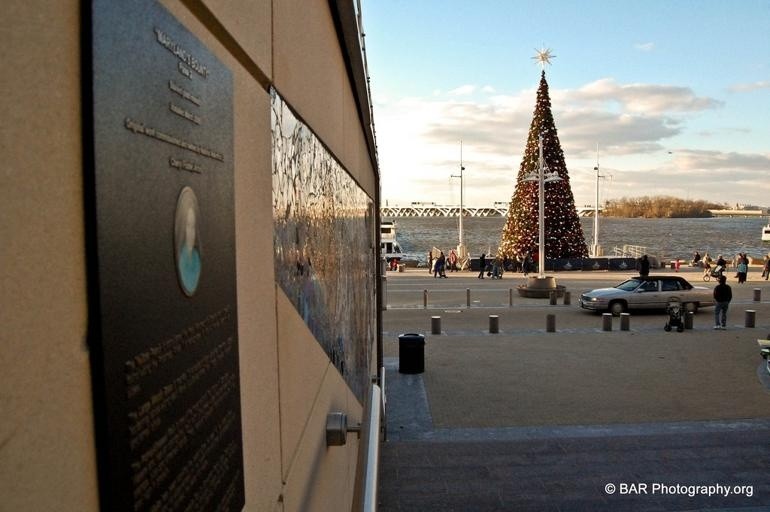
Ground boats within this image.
[761,224,770,241]
[379,221,403,262]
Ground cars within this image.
[579,276,714,316]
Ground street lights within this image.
[594,167,599,244]
[520,136,564,289]
[459,167,465,245]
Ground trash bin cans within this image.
[398,333,425,375]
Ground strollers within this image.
[664,297,683,332]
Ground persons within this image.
[674,251,770,284]
[427,248,540,280]
[639,255,650,276]
[712,275,732,332]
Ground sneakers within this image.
[713,326,727,330]
